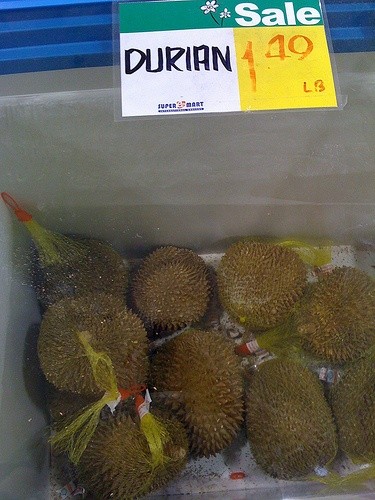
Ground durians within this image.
[34,233,375,500]
